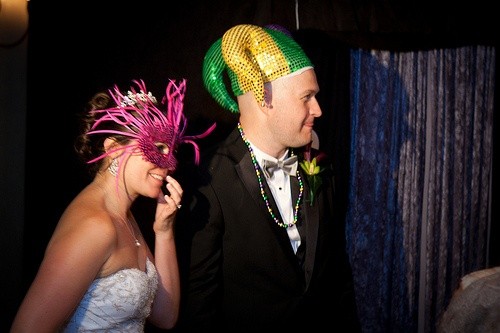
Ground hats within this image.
[202,24,314,113]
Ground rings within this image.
[177,205,182,209]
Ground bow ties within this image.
[263,156,298,178]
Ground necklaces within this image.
[237,123,304,229]
[93,179,141,247]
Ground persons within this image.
[172,24,362,333]
[9,78,216,333]
